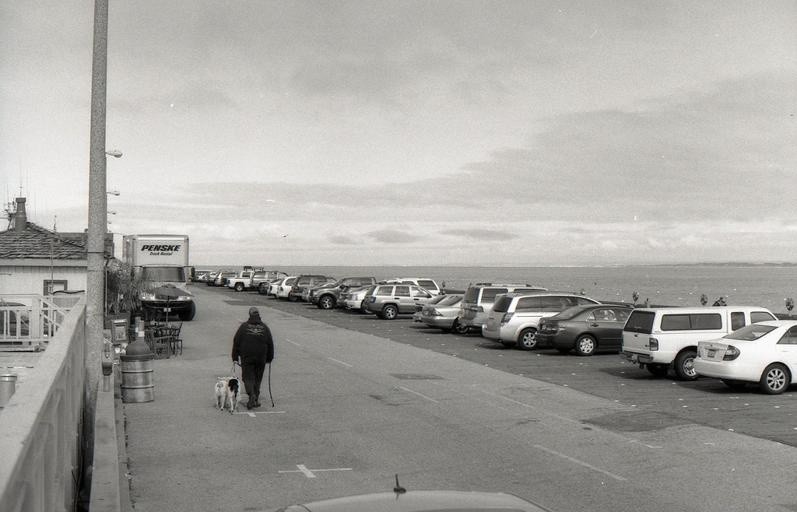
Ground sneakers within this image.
[253,401,260,407]
[247,394,256,409]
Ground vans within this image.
[277,276,297,301]
[291,274,336,300]
[386,278,440,296]
[461,282,549,336]
[622,306,779,380]
[251,271,289,294]
[482,293,602,351]
[363,284,443,319]
[267,279,282,298]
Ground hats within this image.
[248,306,259,316]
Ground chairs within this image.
[127,322,183,358]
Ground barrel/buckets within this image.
[121,352,155,404]
[0,373,18,408]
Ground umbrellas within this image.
[144,283,195,336]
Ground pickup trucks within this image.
[227,270,255,291]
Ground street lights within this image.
[105,147,123,231]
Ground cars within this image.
[336,285,361,308]
[345,285,375,314]
[693,319,797,395]
[279,474,552,511]
[193,270,227,287]
[422,294,468,333]
[536,304,635,355]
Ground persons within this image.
[232,307,274,410]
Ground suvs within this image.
[310,277,375,310]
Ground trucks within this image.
[122,235,195,322]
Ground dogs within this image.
[226,376,240,415]
[214,377,226,412]
